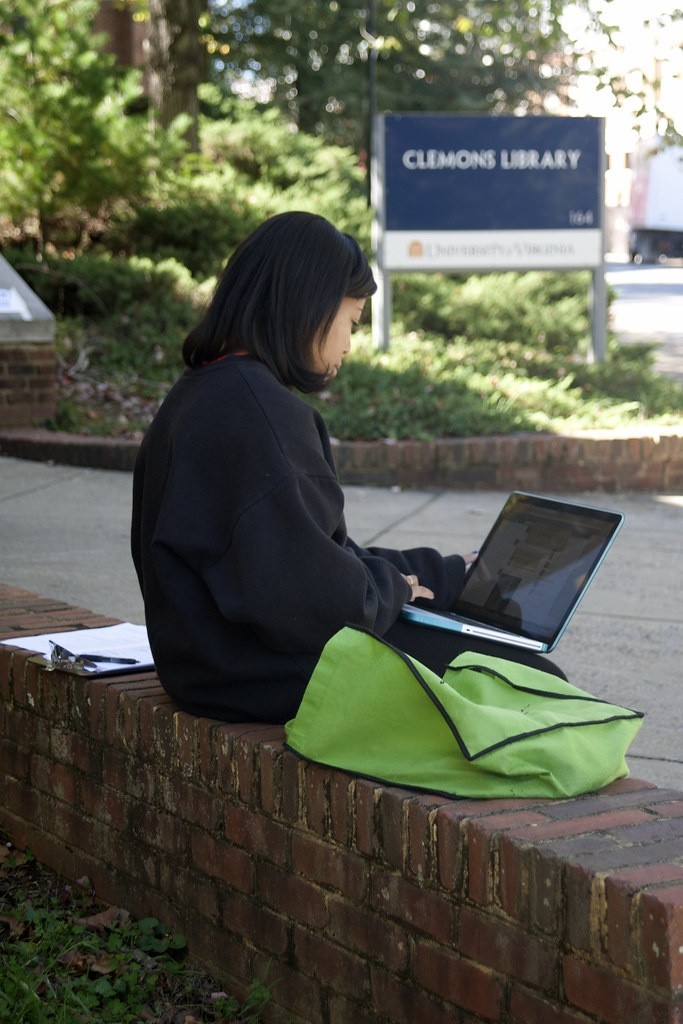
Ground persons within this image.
[129,210,568,725]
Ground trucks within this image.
[626,149,683,265]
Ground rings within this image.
[407,576,413,585]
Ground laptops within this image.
[401,491,624,654]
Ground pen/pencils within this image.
[78,654,140,665]
